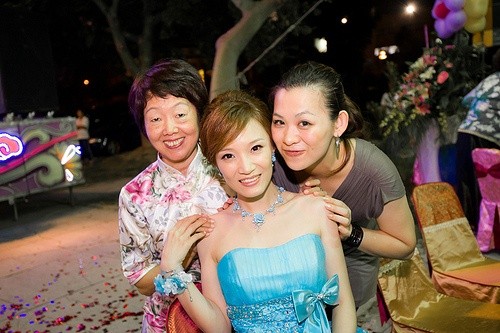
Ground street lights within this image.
[402,2,431,50]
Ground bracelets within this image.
[155,270,194,302]
[343,222,363,248]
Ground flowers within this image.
[376,41,456,136]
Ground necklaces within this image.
[232,187,287,225]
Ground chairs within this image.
[411,182,500,305]
[376,248,500,333]
[471,148,500,252]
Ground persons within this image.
[218,63,416,321]
[119,61,330,333]
[153,89,369,333]
[76,110,93,162]
